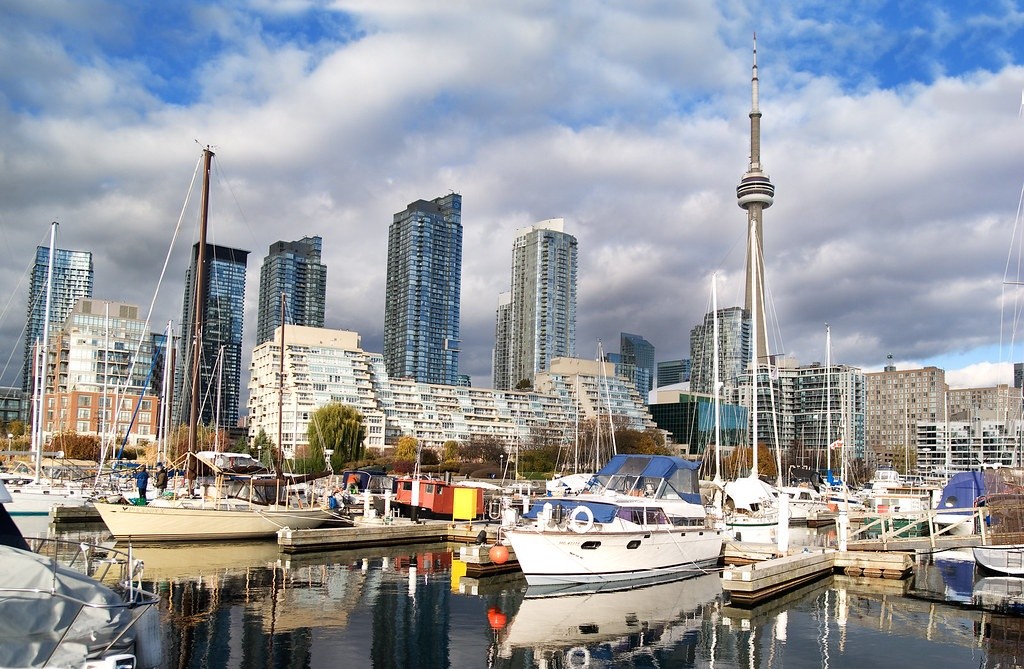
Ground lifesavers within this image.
[569,506,594,535]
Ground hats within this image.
[155,462,165,467]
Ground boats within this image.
[0,28,1024,669]
[501,567,721,653]
[85,536,280,586]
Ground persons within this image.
[154,462,167,498]
[132,465,148,500]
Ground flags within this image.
[830,439,844,451]
